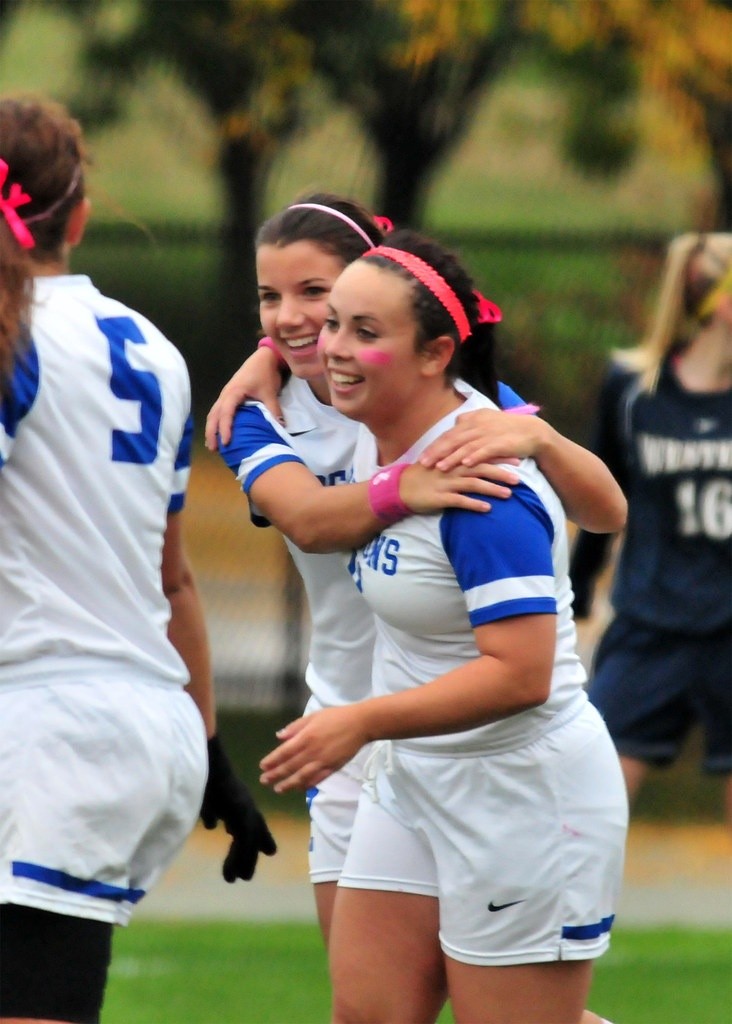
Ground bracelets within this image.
[370,463,415,524]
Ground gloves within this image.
[198,735,275,882]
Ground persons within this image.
[204,230,625,1024]
[213,193,628,1021]
[1,91,279,1022]
[570,232,731,835]
